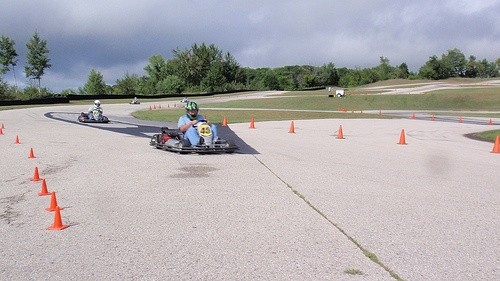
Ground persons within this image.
[184,96,188,101]
[88,100,102,114]
[134,97,137,101]
[178,101,210,135]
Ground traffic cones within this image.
[396,129,409,145]
[0,123,6,135]
[336,107,492,124]
[221,116,228,127]
[489,136,500,154]
[336,125,345,139]
[47,207,69,231]
[37,179,52,196]
[31,167,43,181]
[28,148,37,158]
[14,136,21,144]
[150,104,176,109]
[249,117,255,129]
[287,121,296,133]
[45,192,64,212]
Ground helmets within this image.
[186,101,199,120]
[95,100,101,109]
